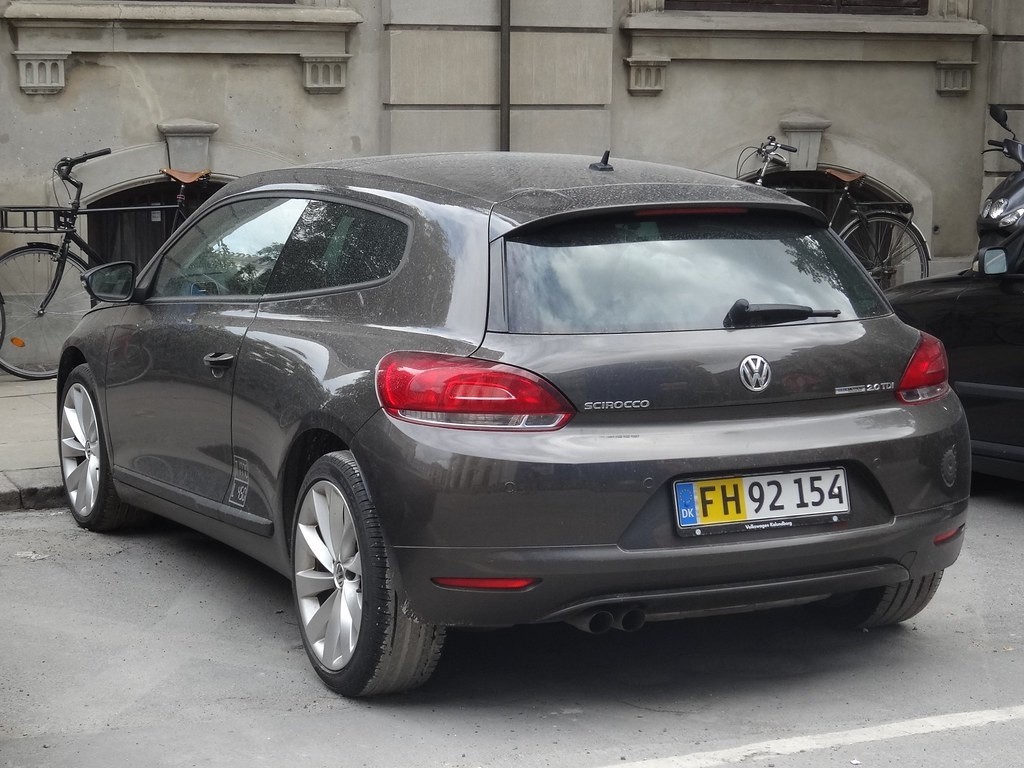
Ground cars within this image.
[880,222,1024,496]
[52,147,974,699]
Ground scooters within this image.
[964,102,1024,275]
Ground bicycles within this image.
[736,135,931,295]
[1,148,211,381]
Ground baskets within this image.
[0,206,75,233]
